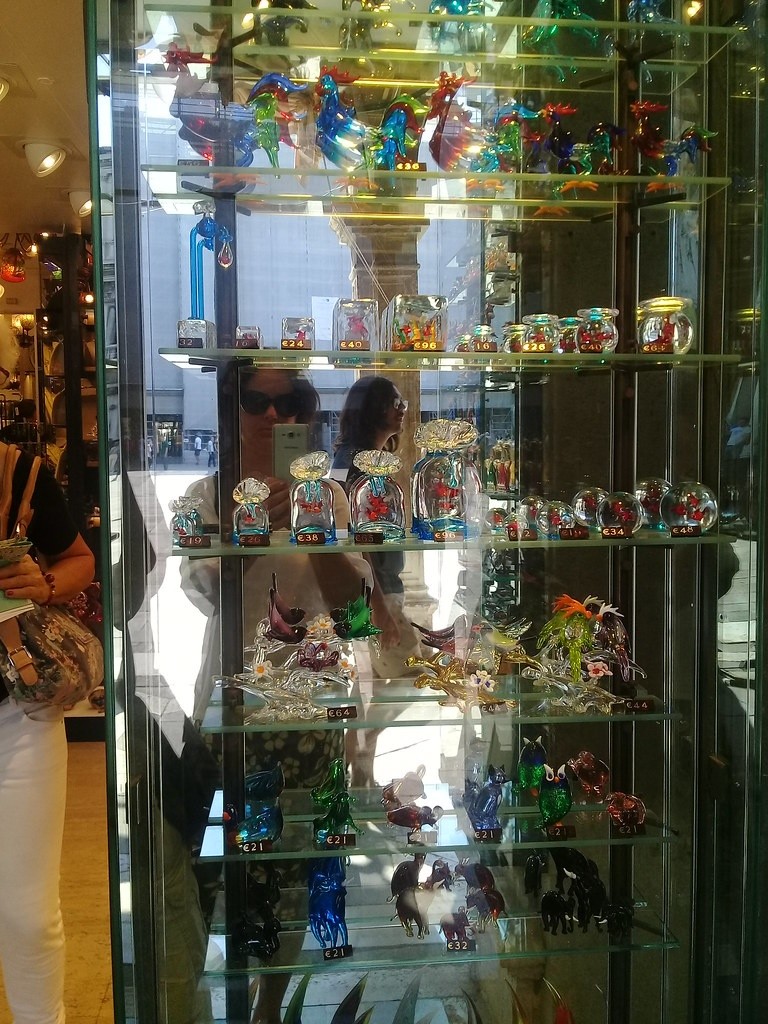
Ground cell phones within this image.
[273,423,310,482]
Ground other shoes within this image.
[347,782,404,811]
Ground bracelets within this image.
[38,570,56,606]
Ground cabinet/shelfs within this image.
[83,0,768,1024]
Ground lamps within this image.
[68,191,92,218]
[24,142,66,180]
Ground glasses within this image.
[239,390,303,417]
[390,397,409,410]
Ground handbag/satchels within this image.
[0,443,104,704]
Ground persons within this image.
[181,348,373,1024]
[146,434,218,470]
[0,438,94,1024]
[329,376,427,820]
